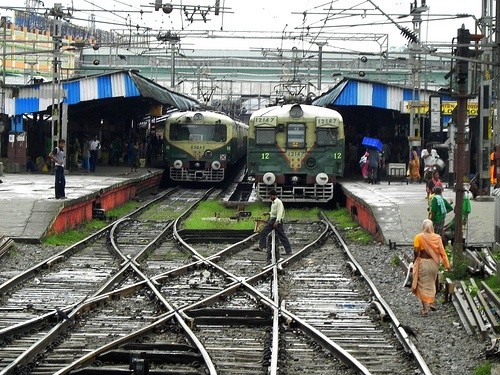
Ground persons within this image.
[252,190,293,255]
[25,134,166,173]
[406,146,422,184]
[48,139,67,199]
[409,218,450,316]
[428,172,471,247]
[421,143,440,181]
[346,135,407,185]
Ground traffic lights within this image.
[445,24,485,277]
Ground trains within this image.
[162,110,248,183]
[247,102,346,202]
